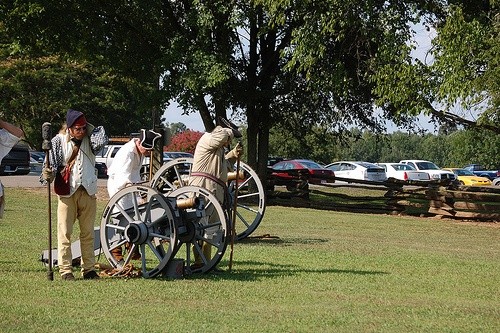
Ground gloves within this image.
[42,168,54,182]
[234,142,243,158]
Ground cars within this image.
[30,151,46,174]
[163,152,193,174]
[324,161,388,184]
[269,160,335,184]
[492,177,500,186]
[442,168,492,187]
[374,163,430,182]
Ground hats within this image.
[65,108,85,127]
[217,115,241,139]
[138,128,162,150]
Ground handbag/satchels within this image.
[54,166,70,196]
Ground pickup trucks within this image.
[460,164,500,181]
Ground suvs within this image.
[399,160,457,185]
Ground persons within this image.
[188,113,245,275]
[39,110,109,281]
[106,128,172,277]
[0,118,24,219]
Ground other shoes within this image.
[62,273,76,281]
[84,270,99,279]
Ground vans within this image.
[0,145,30,176]
[95,145,150,179]
